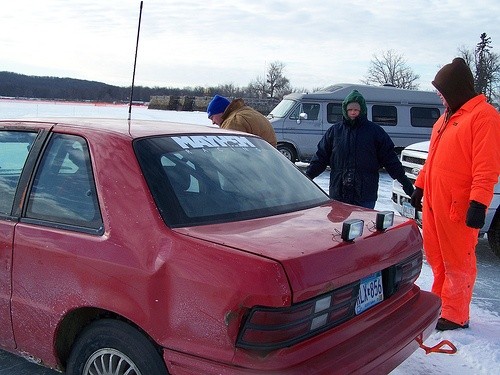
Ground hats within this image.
[207,95,230,118]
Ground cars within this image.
[0,1,443,375]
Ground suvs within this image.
[389,139,500,259]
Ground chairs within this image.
[164,166,218,214]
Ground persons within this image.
[305,90,415,208]
[207,94,277,150]
[410,58,500,331]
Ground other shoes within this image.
[436,317,469,331]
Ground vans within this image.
[264,82,445,168]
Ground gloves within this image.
[465,201,487,229]
[404,183,415,196]
[411,188,423,211]
